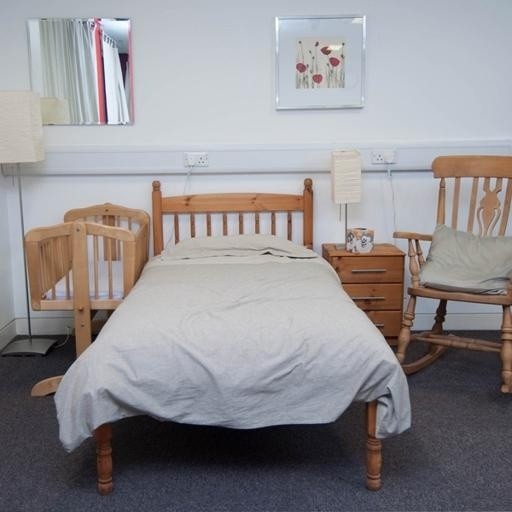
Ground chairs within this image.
[392,156,512,395]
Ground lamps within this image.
[331,147,363,251]
[0,89,59,358]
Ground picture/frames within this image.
[274,14,369,111]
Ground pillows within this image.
[421,222,512,297]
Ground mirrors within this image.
[26,15,136,126]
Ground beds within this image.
[21,197,149,398]
[64,177,405,496]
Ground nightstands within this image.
[320,243,409,352]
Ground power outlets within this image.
[370,150,398,164]
[185,151,209,167]
